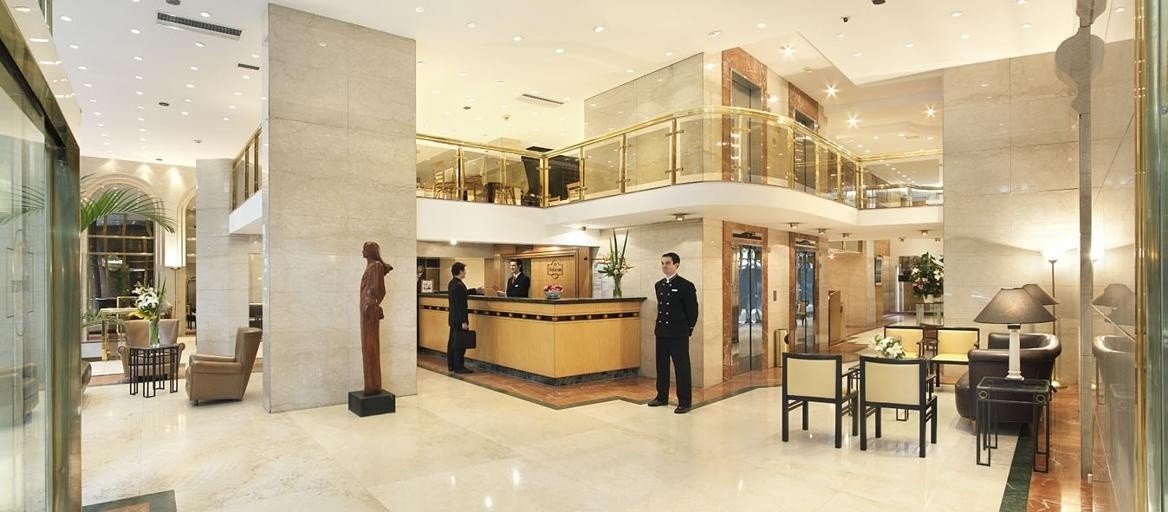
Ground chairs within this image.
[844,323,981,421]
[116,319,185,382]
[794,300,809,326]
[418,157,524,209]
[184,302,196,330]
[181,325,263,406]
[853,355,938,459]
[779,351,859,449]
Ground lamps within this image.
[1020,283,1059,333]
[161,264,188,320]
[972,285,1059,386]
[1091,283,1135,329]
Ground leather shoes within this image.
[675,405,691,413]
[648,399,669,406]
[448,365,473,373]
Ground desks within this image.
[973,374,1051,476]
[913,302,945,328]
[1103,383,1134,479]
[95,307,140,360]
[126,342,181,398]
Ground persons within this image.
[446,262,484,374]
[496,259,530,298]
[358,241,394,396]
[648,251,699,415]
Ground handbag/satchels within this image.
[454,329,476,348]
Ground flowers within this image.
[908,251,944,298]
[542,284,564,292]
[590,223,637,295]
[126,264,170,343]
[870,331,906,363]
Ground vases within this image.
[543,291,562,299]
[147,317,161,349]
[920,292,934,304]
[611,274,624,299]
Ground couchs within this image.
[1089,333,1136,388]
[953,329,1062,440]
